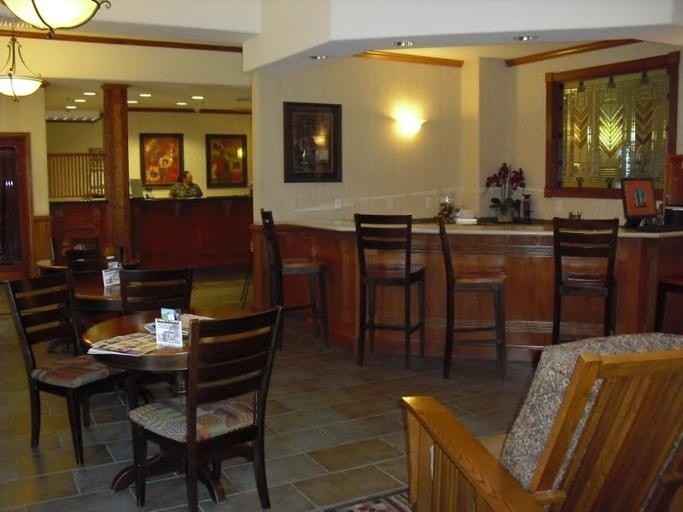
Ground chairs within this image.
[257,206,331,351]
[398,331,681,512]
[351,212,427,368]
[549,215,620,346]
[125,305,282,512]
[647,275,682,335]
[33,232,195,314]
[1,267,138,468]
[435,213,507,381]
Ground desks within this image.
[78,304,284,504]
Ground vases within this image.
[495,206,515,223]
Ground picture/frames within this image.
[282,100,342,184]
[204,133,248,189]
[138,132,185,191]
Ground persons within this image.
[169,170,203,197]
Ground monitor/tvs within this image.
[621,177,657,229]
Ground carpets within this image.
[309,486,413,512]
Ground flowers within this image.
[482,161,526,213]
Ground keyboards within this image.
[641,225,682,231]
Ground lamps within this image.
[0,0,111,40]
[0,26,43,103]
